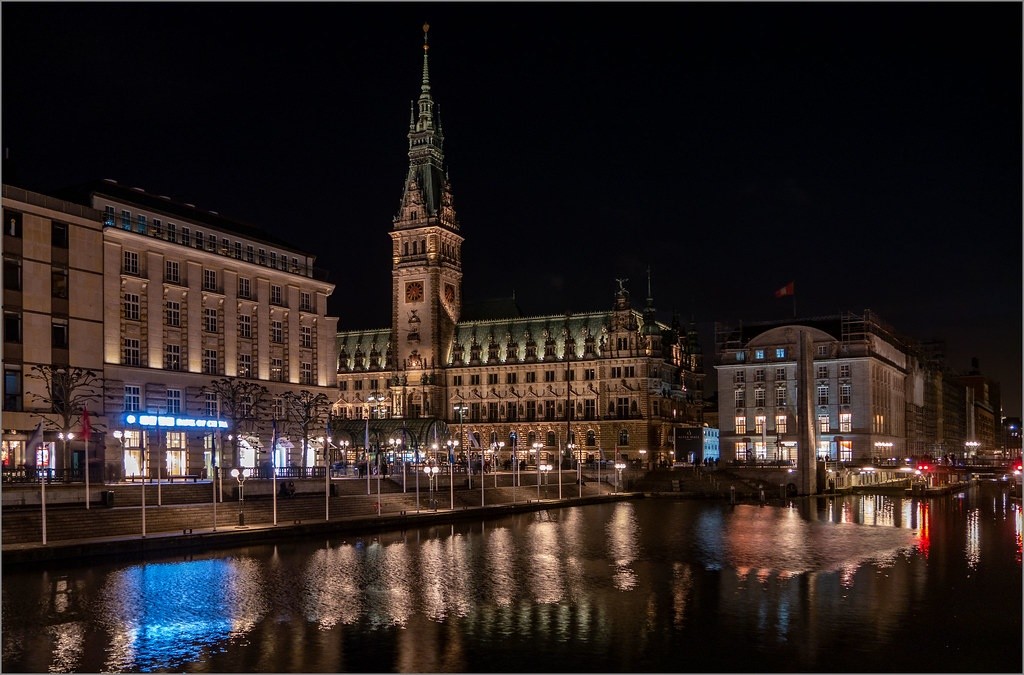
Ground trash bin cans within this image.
[100,490,114,507]
[328,482,339,497]
[232,486,242,501]
[464,478,474,489]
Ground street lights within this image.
[493,441,505,471]
[539,464,553,500]
[340,440,350,476]
[615,463,627,494]
[423,466,439,512]
[57,431,75,482]
[113,430,133,484]
[533,443,544,473]
[389,438,402,475]
[447,440,459,455]
[231,468,249,529]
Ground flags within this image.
[470,431,479,449]
[80,405,92,439]
[26,421,43,466]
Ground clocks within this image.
[404,281,425,304]
[443,280,456,306]
